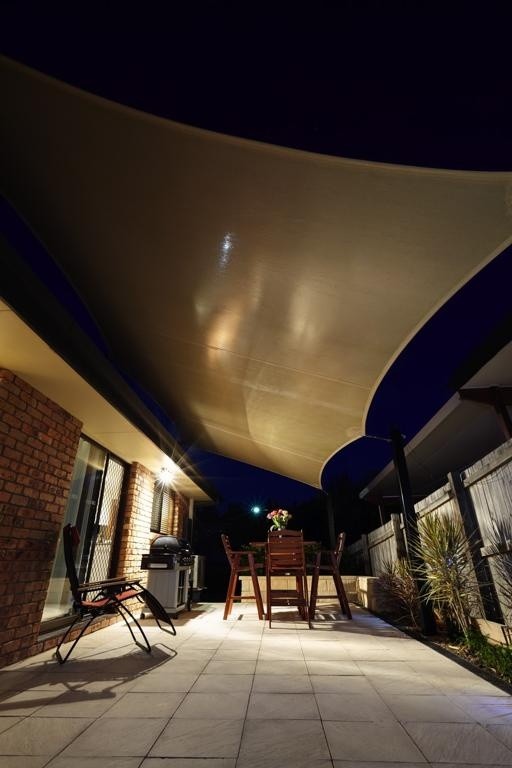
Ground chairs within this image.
[220,532,352,629]
[55,523,176,665]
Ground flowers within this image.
[267,509,292,532]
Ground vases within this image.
[277,528,285,538]
